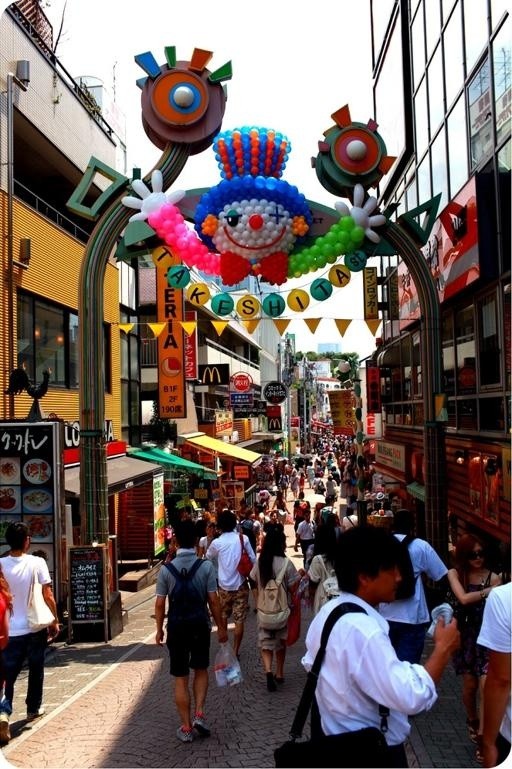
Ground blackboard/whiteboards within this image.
[66,543,108,624]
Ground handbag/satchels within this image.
[27,558,56,631]
[274,726,391,769]
[237,534,253,577]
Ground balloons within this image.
[120,125,387,319]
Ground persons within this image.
[0,563,14,701]
[156,432,512,767]
[0,521,60,745]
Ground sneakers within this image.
[176,725,193,742]
[473,743,484,763]
[192,712,211,738]
[466,717,480,744]
[0,711,10,741]
[26,708,45,721]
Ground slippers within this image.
[274,674,284,682]
[266,671,277,692]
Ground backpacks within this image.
[317,554,340,596]
[165,559,208,632]
[257,557,291,630]
[395,534,423,600]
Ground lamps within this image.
[453,450,465,466]
[484,456,499,476]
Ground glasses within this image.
[466,549,485,560]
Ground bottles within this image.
[213,664,242,689]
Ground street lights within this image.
[335,359,364,500]
